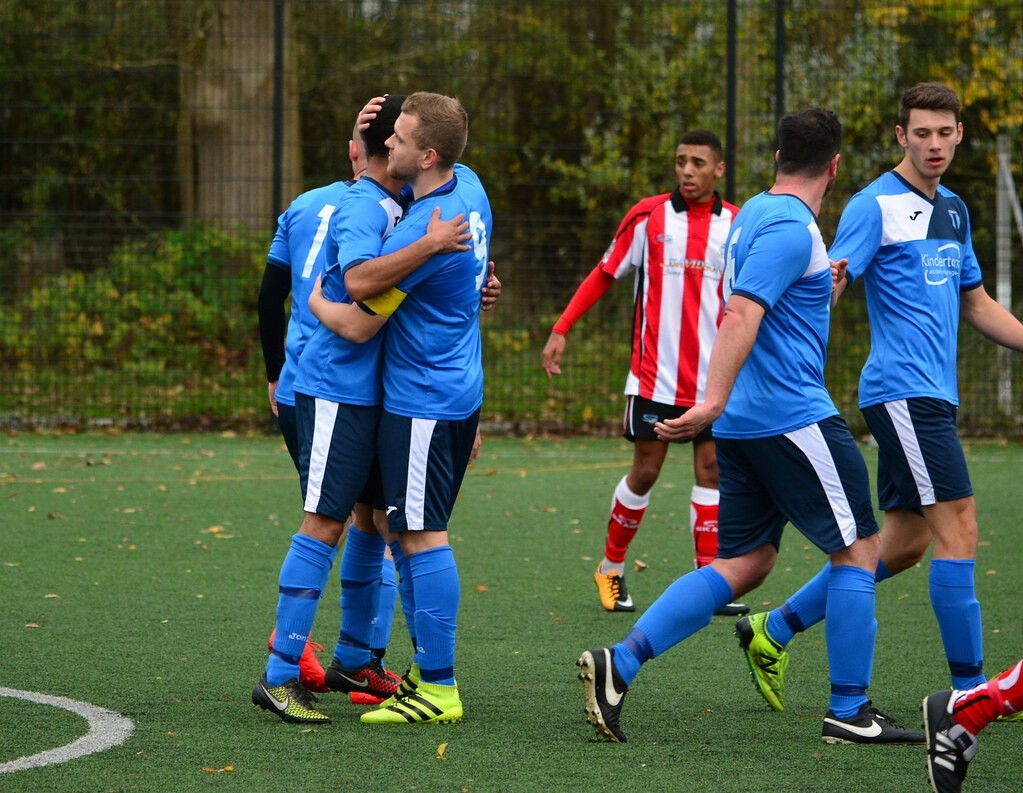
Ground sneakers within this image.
[251,671,332,725]
[819,700,926,745]
[922,689,978,793]
[591,560,636,612]
[576,646,627,742]
[377,662,422,708]
[733,609,789,713]
[325,653,404,698]
[267,626,332,693]
[949,685,1023,722]
[713,601,751,617]
[358,680,466,725]
[350,690,389,705]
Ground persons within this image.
[736,81,1022,722]
[576,104,926,745]
[540,129,751,615]
[920,657,1022,793]
[250,93,406,723]
[356,88,495,724]
[260,122,404,692]
